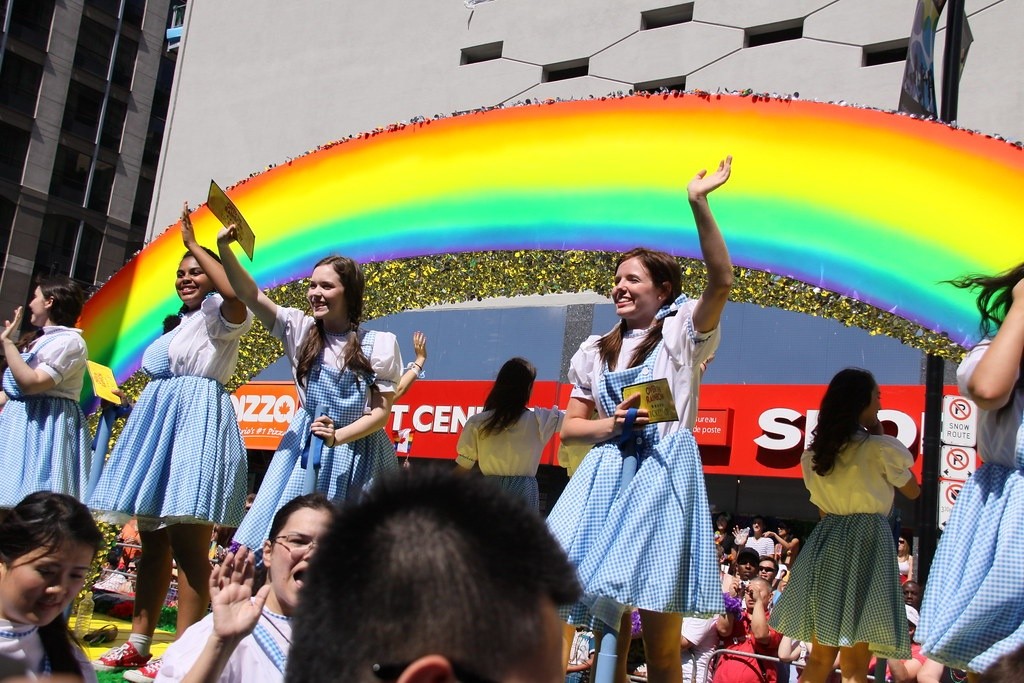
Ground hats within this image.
[737,548,760,562]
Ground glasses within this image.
[274,534,313,550]
[759,566,775,572]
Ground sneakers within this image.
[90,641,152,671]
[123,658,164,682]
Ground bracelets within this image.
[326,436,335,447]
[405,361,421,376]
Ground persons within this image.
[0,273,88,507]
[149,493,340,683]
[217,223,425,571]
[0,490,100,683]
[86,199,246,682]
[283,461,583,683]
[89,508,944,682]
[767,368,910,682]
[544,156,732,683]
[913,261,1024,683]
[451,357,566,517]
[388,330,426,402]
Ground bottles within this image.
[73,591,96,639]
[734,527,750,545]
